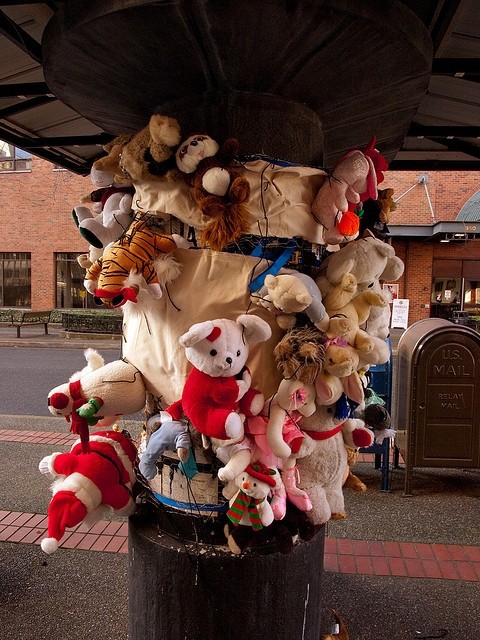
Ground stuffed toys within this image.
[47,347,148,454]
[137,407,190,480]
[312,135,396,245]
[224,461,276,556]
[351,388,397,445]
[200,411,265,499]
[82,211,192,309]
[247,377,316,521]
[161,314,273,443]
[315,237,405,366]
[90,113,187,189]
[38,425,138,555]
[319,274,388,378]
[249,265,330,333]
[331,444,368,521]
[291,395,375,526]
[356,370,373,398]
[172,131,255,251]
[273,325,327,385]
[72,192,136,269]
[81,187,136,214]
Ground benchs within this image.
[7,311,53,338]
